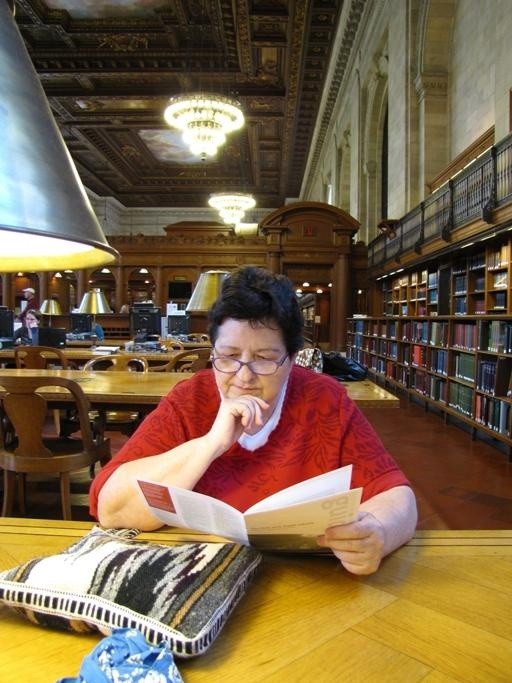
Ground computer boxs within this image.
[125,341,160,353]
[65,333,91,340]
[167,334,187,342]
[0,340,14,350]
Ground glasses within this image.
[208,347,292,375]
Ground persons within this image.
[89,264,417,576]
[13,309,42,346]
[15,288,39,322]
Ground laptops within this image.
[39,328,66,349]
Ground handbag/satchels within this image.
[322,351,368,382]
[1,524,262,657]
[295,348,322,374]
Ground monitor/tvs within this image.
[71,314,91,334]
[129,307,161,343]
[0,310,14,337]
[168,315,188,335]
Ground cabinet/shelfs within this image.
[345,218,512,464]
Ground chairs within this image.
[1,374,112,521]
[0,345,212,521]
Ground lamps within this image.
[40,299,62,328]
[185,270,231,312]
[163,14,258,225]
[79,291,114,350]
[0,1,122,275]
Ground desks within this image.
[0,516,512,683]
[0,339,402,409]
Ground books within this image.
[93,345,121,355]
[344,245,512,439]
